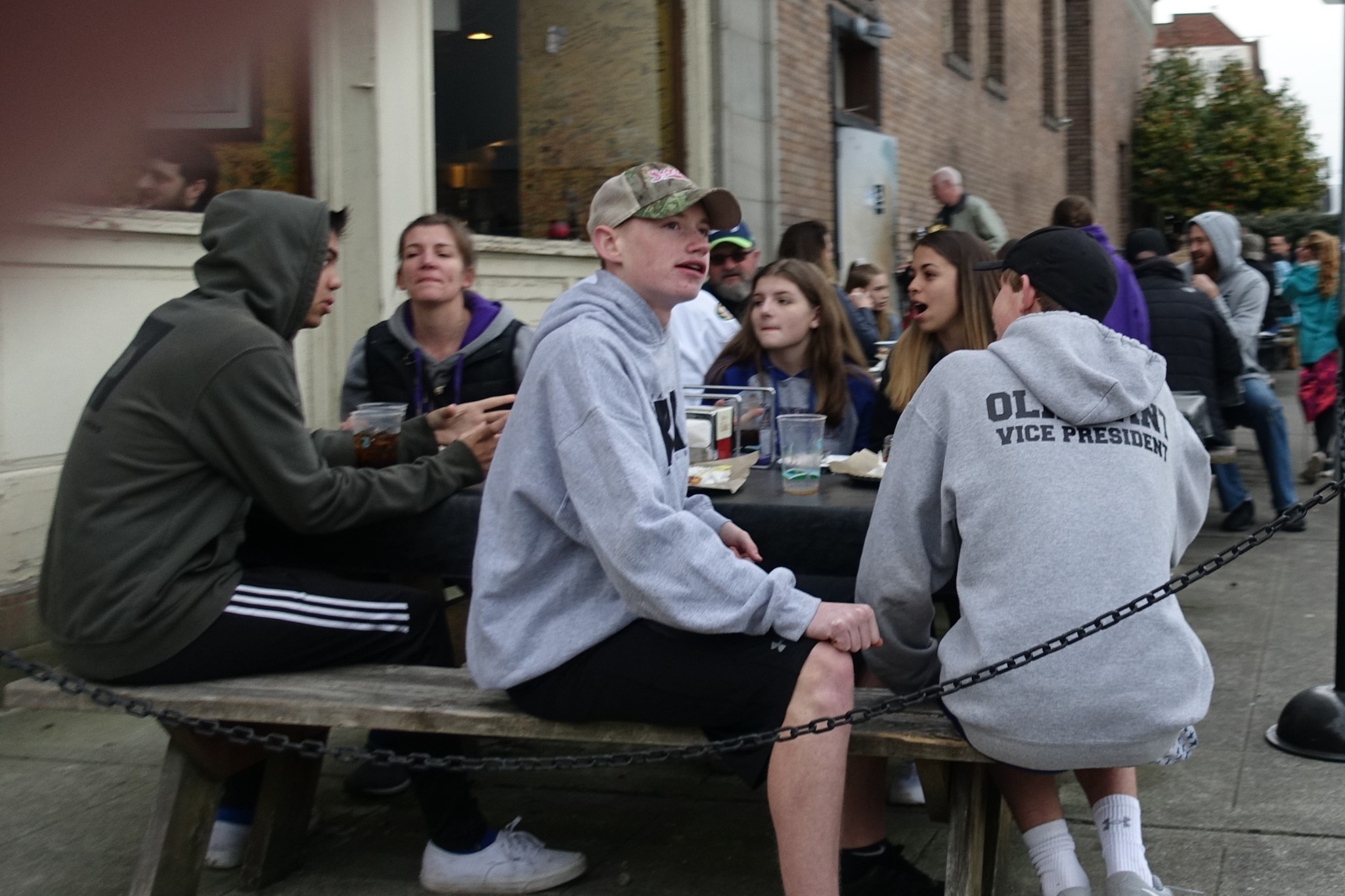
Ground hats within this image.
[586,161,742,239]
[972,226,1118,320]
[1123,229,1168,258]
[1241,234,1264,260]
[706,220,756,249]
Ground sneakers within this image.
[1279,506,1309,532]
[204,820,248,869]
[420,817,586,894]
[885,761,925,804]
[1228,500,1255,530]
[1302,450,1326,483]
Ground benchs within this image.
[15,641,1007,766]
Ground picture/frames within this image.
[151,40,263,138]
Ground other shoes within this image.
[846,845,946,896]
[1109,871,1204,896]
[1060,886,1091,896]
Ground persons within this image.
[36,188,587,896]
[766,230,1002,861]
[339,211,534,795]
[1166,233,1192,266]
[845,260,902,341]
[1176,210,1307,532]
[1243,228,1340,482]
[666,288,743,405]
[133,136,218,212]
[928,167,1007,252]
[699,258,877,452]
[701,222,760,322]
[463,162,882,896]
[854,226,1216,896]
[1053,197,1151,346]
[1123,227,1243,448]
[776,219,881,360]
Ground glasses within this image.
[712,248,752,266]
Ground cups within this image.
[359,402,408,417]
[777,413,828,495]
[349,410,403,469]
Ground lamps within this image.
[849,17,897,40]
[1055,117,1073,133]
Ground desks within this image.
[74,434,1004,896]
[868,361,1209,440]
